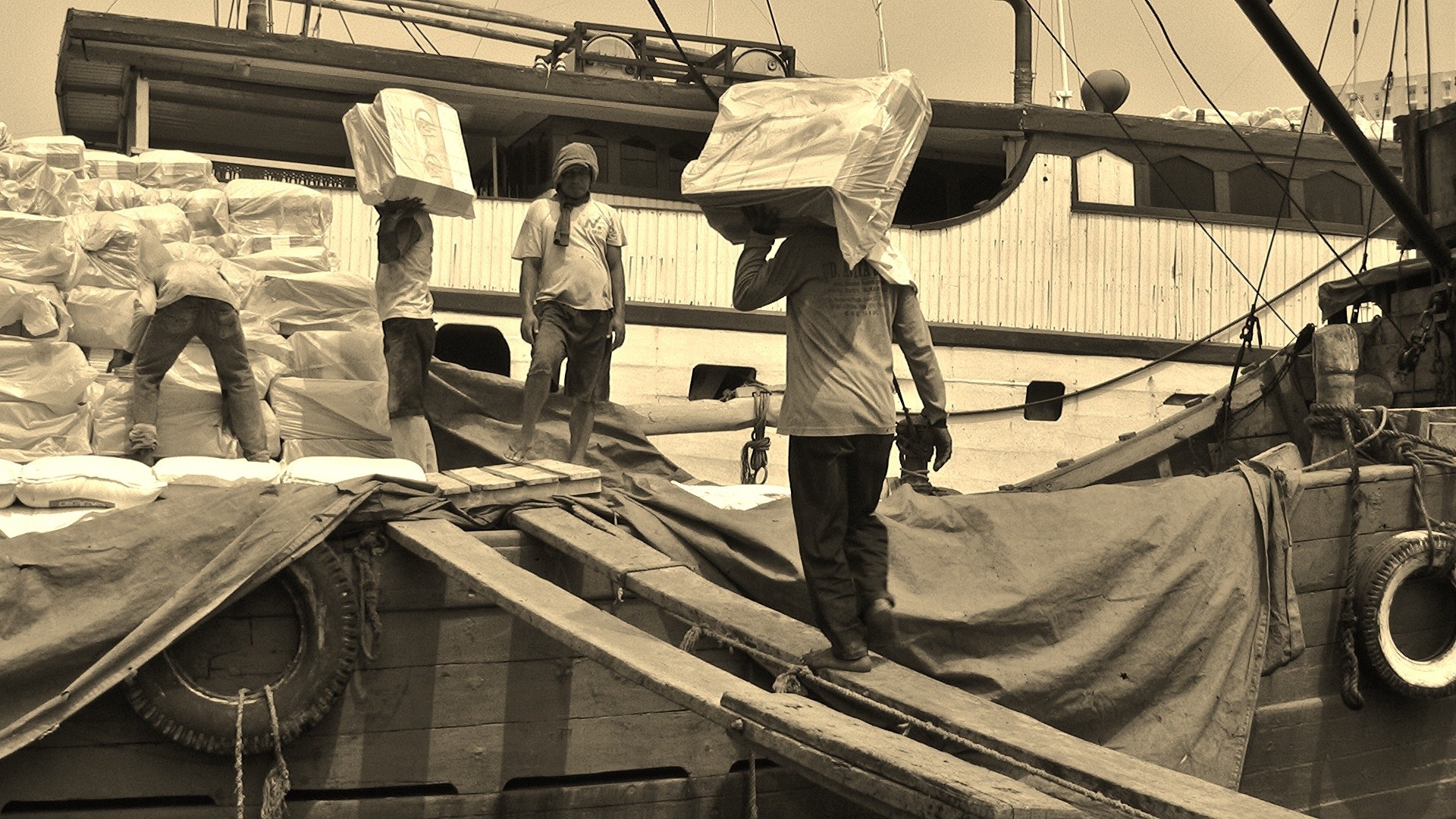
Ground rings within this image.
[113,361,115,362]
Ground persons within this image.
[733,205,953,673]
[374,195,438,473]
[502,142,627,465]
[107,260,270,466]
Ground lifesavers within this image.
[121,538,358,761]
[1353,528,1456,701]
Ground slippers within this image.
[503,429,536,463]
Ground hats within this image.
[562,165,591,173]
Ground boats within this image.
[51,0,1420,496]
[0,0,1456,819]
